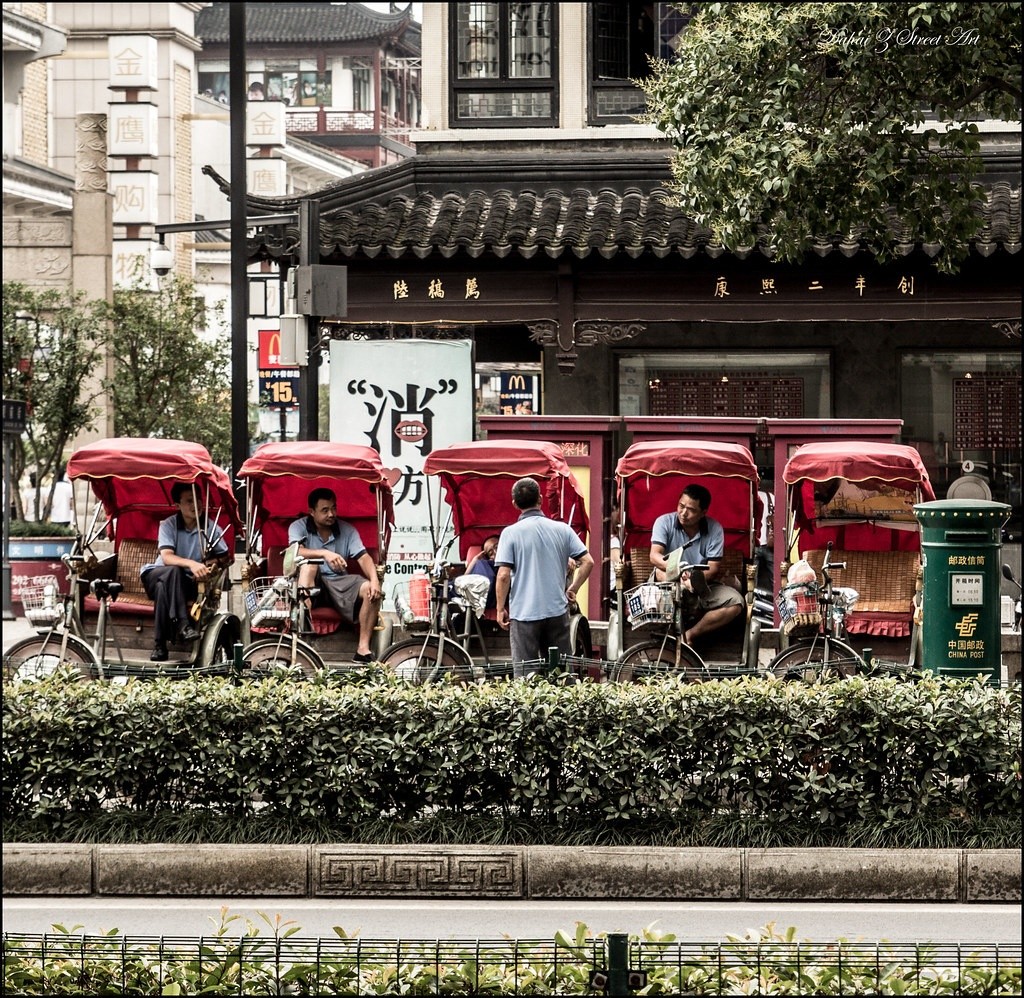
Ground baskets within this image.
[392,578,432,632]
[19,575,60,627]
[623,581,675,631]
[242,576,290,628]
[774,582,820,637]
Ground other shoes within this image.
[180,626,201,640]
[150,643,169,661]
[303,616,313,632]
[689,569,712,600]
[352,651,376,663]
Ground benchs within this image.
[467,545,568,621]
[630,545,744,619]
[795,550,923,637]
[83,537,225,616]
[267,544,380,620]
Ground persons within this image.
[649,483,745,651]
[465,534,500,575]
[287,487,381,664]
[139,481,229,661]
[494,476,596,682]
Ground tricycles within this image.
[375,440,592,697]
[609,440,762,696]
[235,441,394,678]
[3,438,241,689]
[768,441,936,688]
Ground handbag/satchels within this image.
[787,559,817,584]
